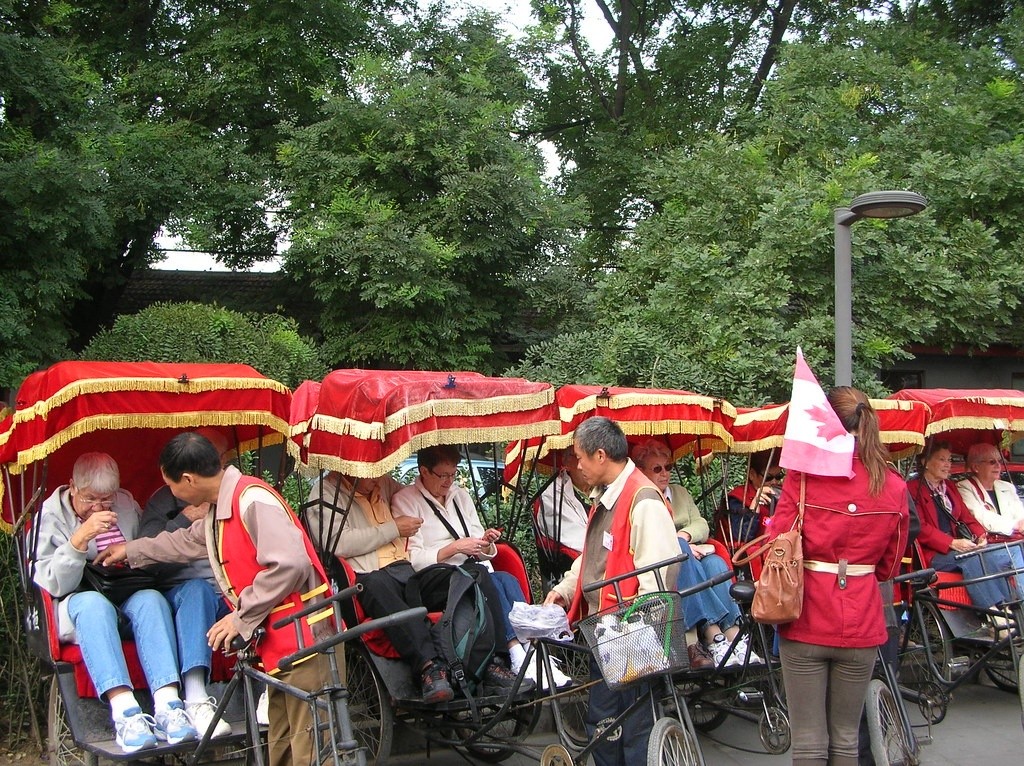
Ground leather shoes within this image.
[689,642,715,669]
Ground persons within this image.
[542,414,682,766]
[537,435,765,668]
[27,450,197,754]
[90,431,349,766]
[305,436,536,701]
[722,445,785,543]
[905,441,1024,641]
[758,386,910,766]
[139,446,271,737]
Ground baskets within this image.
[576,591,691,690]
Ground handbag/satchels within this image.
[956,520,973,540]
[733,532,803,624]
[82,561,157,600]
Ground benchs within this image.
[19,516,260,694]
[333,531,531,680]
[713,500,767,589]
[524,492,736,595]
[908,545,973,610]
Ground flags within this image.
[779,348,855,476]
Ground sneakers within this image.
[707,637,740,668]
[481,661,536,696]
[256,692,269,724]
[730,634,761,664]
[511,655,573,688]
[154,699,198,743]
[422,660,454,704]
[184,696,232,739]
[115,706,159,752]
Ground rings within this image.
[479,544,481,547]
[104,523,109,529]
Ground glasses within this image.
[759,471,786,482]
[431,469,460,480]
[76,486,118,506]
[981,459,1003,465]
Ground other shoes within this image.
[987,610,1015,640]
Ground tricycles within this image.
[1,362,428,766]
[881,388,1024,693]
[696,398,1023,726]
[505,383,943,766]
[287,369,739,766]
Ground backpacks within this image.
[406,562,495,694]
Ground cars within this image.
[310,459,510,514]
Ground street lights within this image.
[833,191,928,387]
